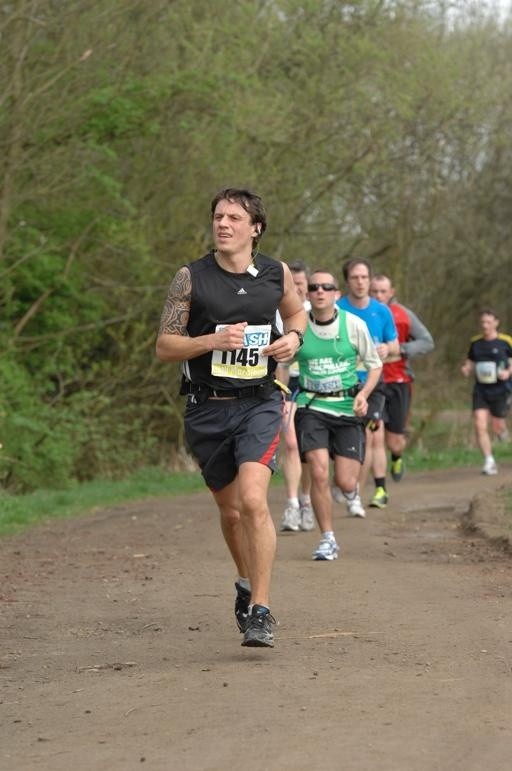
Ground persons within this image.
[276,264,315,531]
[460,307,512,475]
[277,268,383,563]
[155,187,309,650]
[368,274,437,507]
[333,257,401,510]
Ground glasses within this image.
[307,284,338,292]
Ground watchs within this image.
[287,327,304,347]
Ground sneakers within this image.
[312,535,341,562]
[241,603,278,649]
[368,488,390,509]
[347,498,366,518]
[390,456,404,483]
[330,485,343,505]
[343,491,358,500]
[280,503,300,532]
[299,502,316,532]
[480,461,499,477]
[233,581,253,633]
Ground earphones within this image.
[255,226,262,236]
[334,295,338,301]
